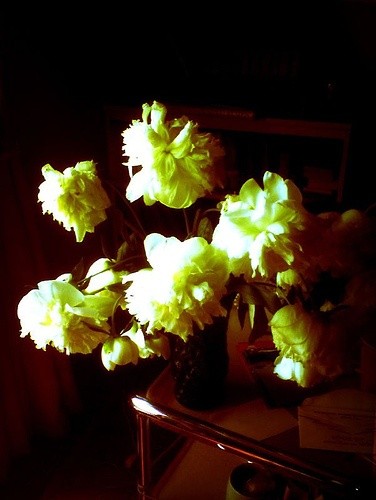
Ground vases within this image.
[170,285,238,410]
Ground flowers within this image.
[17,98,371,388]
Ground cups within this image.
[226,463,278,500]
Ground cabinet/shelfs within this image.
[124,338,376,500]
